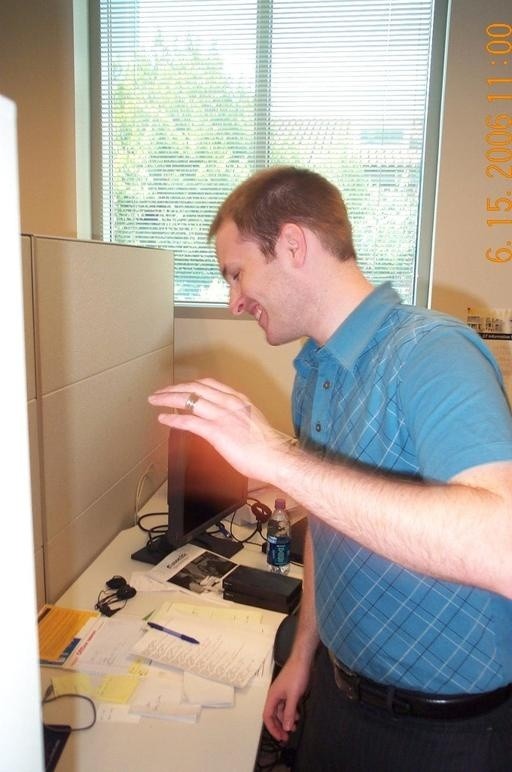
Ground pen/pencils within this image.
[147,622,200,643]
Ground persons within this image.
[172,560,237,593]
[148,167,512,772]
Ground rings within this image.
[185,393,199,413]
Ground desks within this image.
[38,477,304,771]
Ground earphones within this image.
[105,574,127,589]
[117,583,136,601]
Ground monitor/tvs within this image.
[129,422,248,566]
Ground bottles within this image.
[266,497,291,576]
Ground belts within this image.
[327,650,512,722]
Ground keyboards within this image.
[263,516,309,566]
